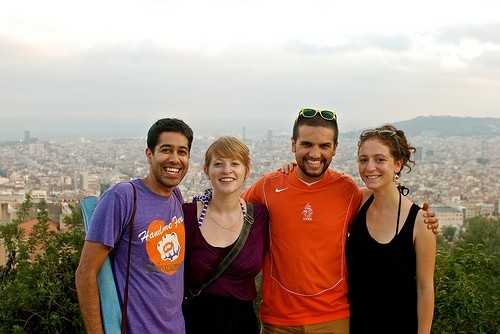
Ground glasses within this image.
[295,109,337,125]
[362,129,401,157]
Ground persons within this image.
[239,109,440,334]
[74,118,194,334]
[277,124,438,334]
[181,136,271,334]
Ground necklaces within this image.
[208,200,241,231]
